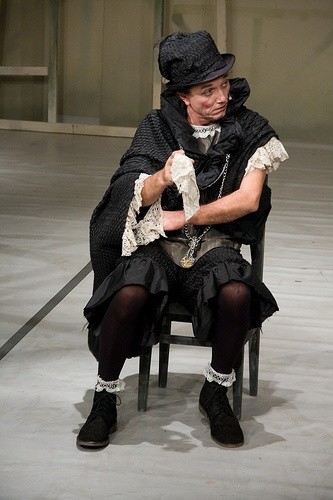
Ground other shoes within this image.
[77,391,121,448]
[198,379,245,448]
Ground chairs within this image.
[138,173,268,420]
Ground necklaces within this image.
[191,122,219,133]
[178,155,231,268]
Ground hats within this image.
[157,31,237,90]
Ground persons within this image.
[76,30,289,449]
